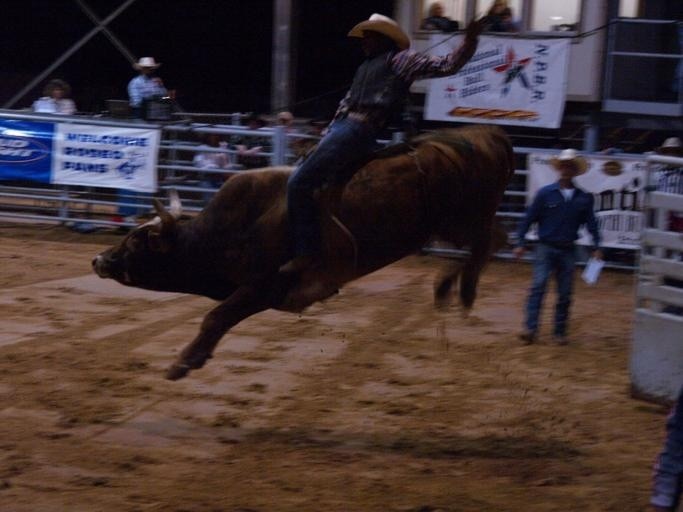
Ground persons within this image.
[228,111,273,170]
[277,109,301,161]
[276,10,487,282]
[651,385,681,512]
[31,78,78,120]
[194,123,232,211]
[126,55,167,119]
[508,146,601,346]
[475,0,518,33]
[644,136,683,197]
[419,4,459,34]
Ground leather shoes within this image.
[516,327,569,344]
[280,253,311,274]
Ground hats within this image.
[654,137,682,151]
[133,57,160,70]
[241,110,294,127]
[347,13,410,50]
[547,149,587,176]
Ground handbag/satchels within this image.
[145,101,171,120]
[33,98,60,114]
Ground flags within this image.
[0,115,53,185]
[422,31,570,131]
[48,120,162,196]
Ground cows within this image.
[90,123,516,382]
